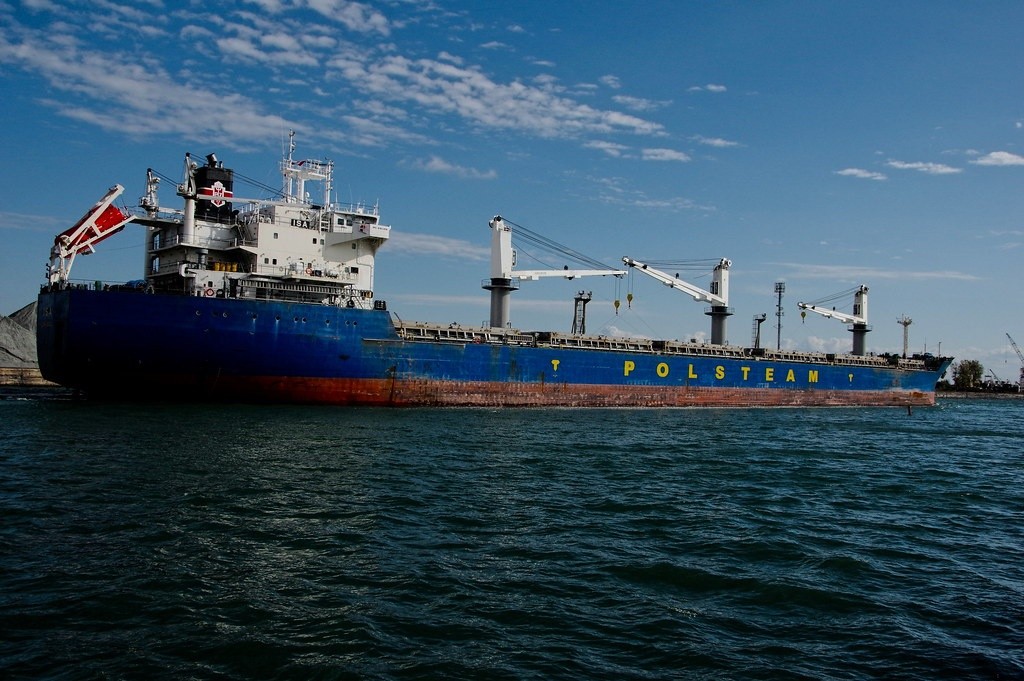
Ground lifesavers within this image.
[206,288,214,296]
[306,268,312,274]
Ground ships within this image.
[31,128,956,410]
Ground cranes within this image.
[1006,332,1023,370]
